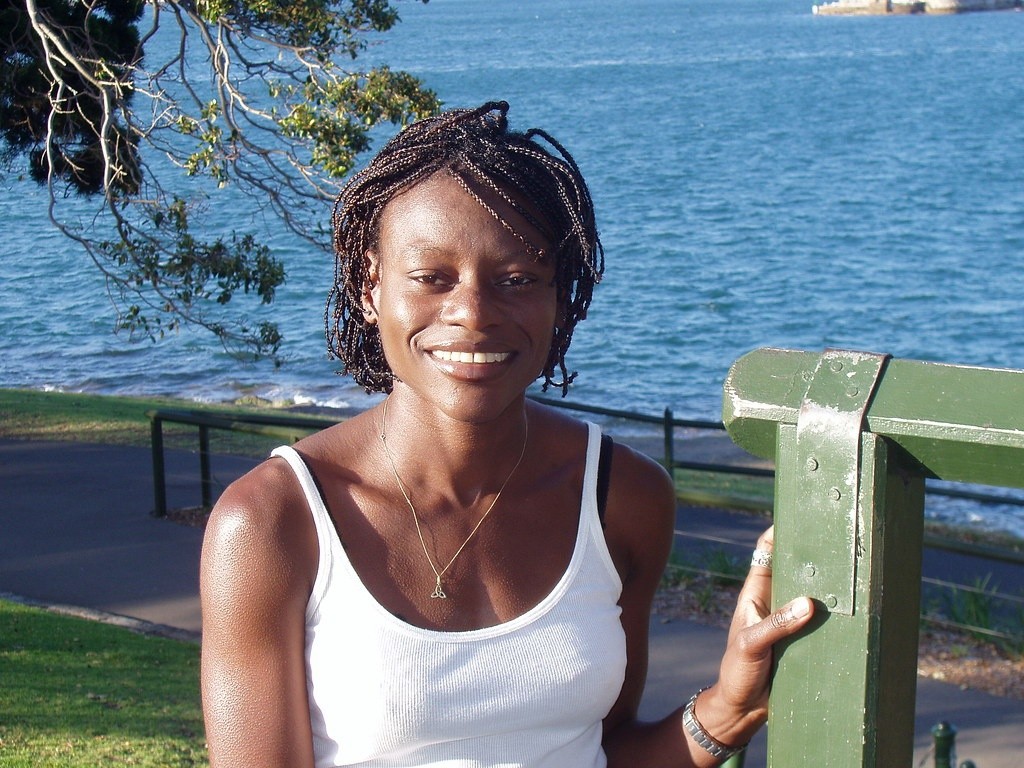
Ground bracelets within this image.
[683,686,751,757]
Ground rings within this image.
[750,549,772,569]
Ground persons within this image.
[199,101,813,768]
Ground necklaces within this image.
[381,393,528,598]
